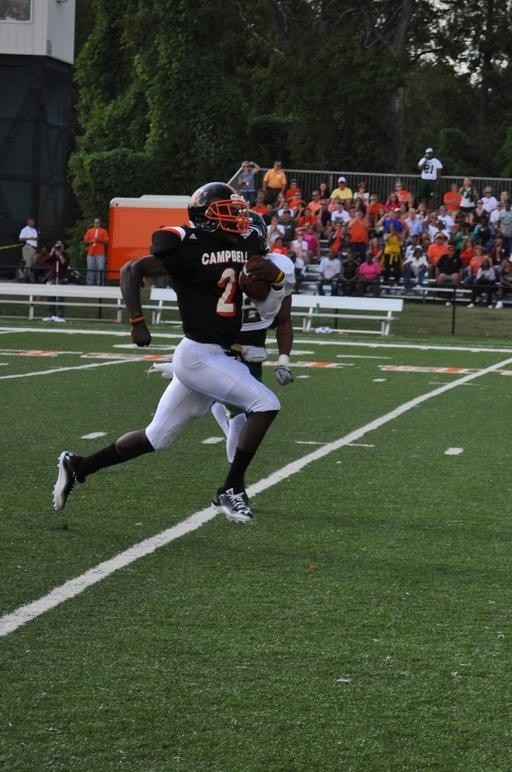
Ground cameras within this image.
[55,245,61,250]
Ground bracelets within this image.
[129,316,146,325]
[273,270,283,282]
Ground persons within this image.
[41,241,70,323]
[221,145,511,313]
[146,208,298,506]
[53,181,287,527]
[15,217,40,279]
[82,217,110,289]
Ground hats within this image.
[338,177,346,183]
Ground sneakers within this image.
[211,487,254,524]
[52,450,86,510]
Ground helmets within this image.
[188,182,267,255]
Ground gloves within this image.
[274,366,294,386]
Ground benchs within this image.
[247,224,510,305]
[1,280,405,336]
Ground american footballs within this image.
[239,255,270,301]
